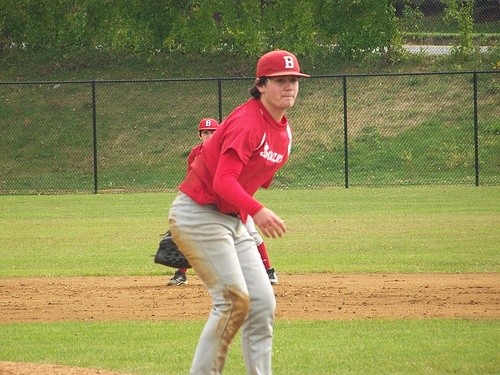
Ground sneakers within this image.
[267,268,278,283]
[168,270,188,286]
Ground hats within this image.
[198,118,219,130]
[256,49,310,78]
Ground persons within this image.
[167,49,311,375]
[167,118,277,285]
